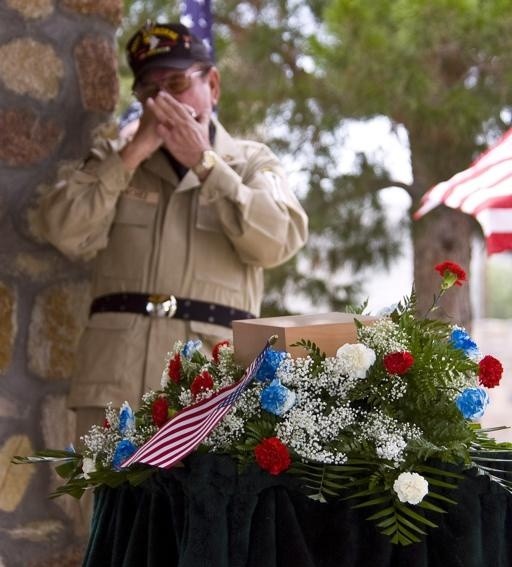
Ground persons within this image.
[38,20,309,532]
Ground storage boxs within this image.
[231,311,391,366]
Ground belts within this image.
[88,292,257,329]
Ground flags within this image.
[412,127,512,254]
[116,342,270,472]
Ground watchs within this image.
[192,149,216,175]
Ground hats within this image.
[126,24,213,89]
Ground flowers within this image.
[6,260,512,543]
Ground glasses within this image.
[132,68,206,103]
